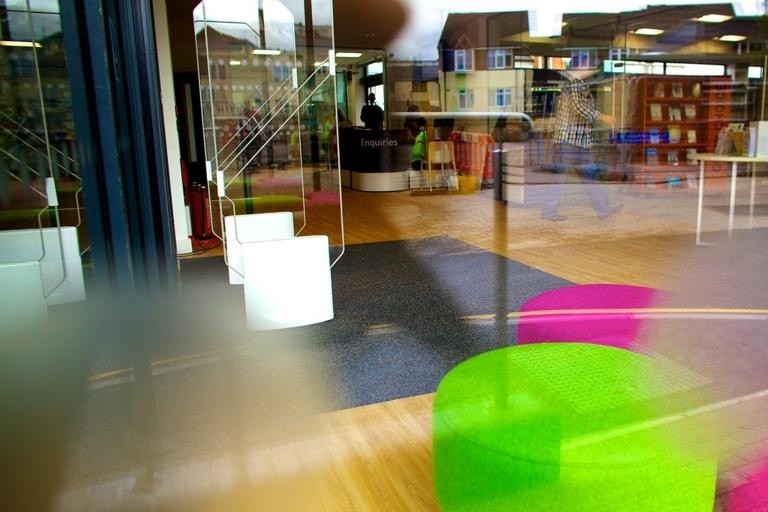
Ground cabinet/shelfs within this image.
[628,71,737,195]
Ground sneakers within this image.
[541,212,566,223]
[599,202,623,220]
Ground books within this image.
[644,81,698,190]
[715,119,768,159]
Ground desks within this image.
[684,148,767,249]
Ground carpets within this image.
[3,232,584,420]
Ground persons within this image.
[360,93,385,131]
[538,54,626,220]
[402,105,430,141]
[519,117,533,141]
[492,114,509,143]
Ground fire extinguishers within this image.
[190,181,212,240]
